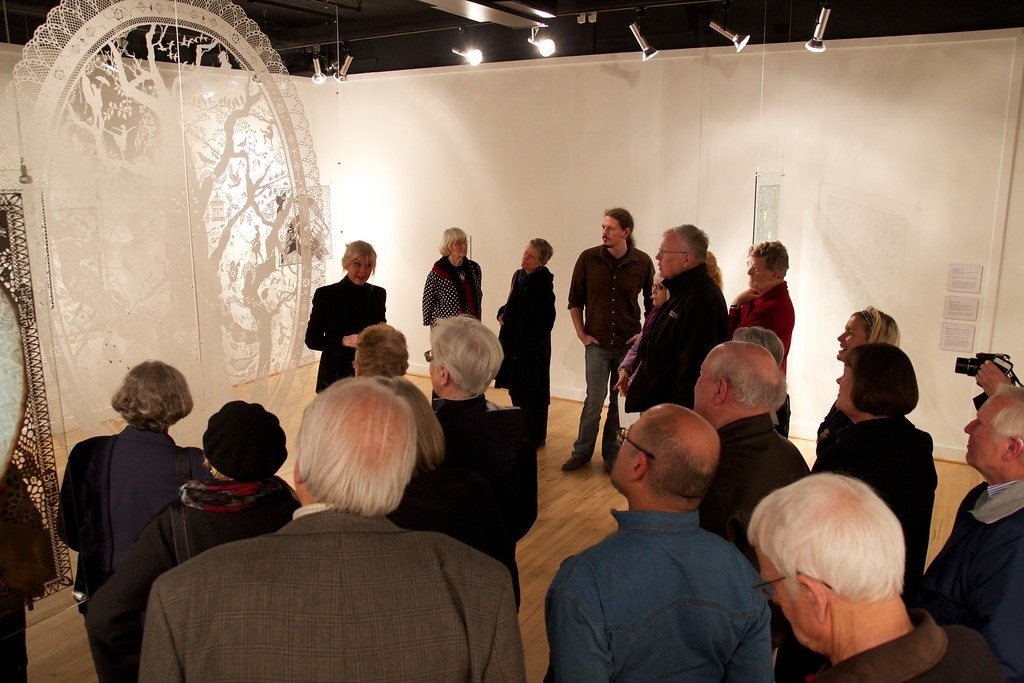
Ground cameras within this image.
[954,353,1004,376]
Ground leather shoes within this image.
[605,459,612,472]
[562,459,591,470]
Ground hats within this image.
[202,400,288,482]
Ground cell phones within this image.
[977,355,1013,386]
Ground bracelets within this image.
[629,377,632,382]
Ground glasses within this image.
[753,572,831,600]
[616,427,655,459]
[747,262,759,274]
[424,350,433,362]
[659,248,688,256]
[456,241,467,247]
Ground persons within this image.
[721,241,796,380]
[494,237,557,448]
[0,306,1024,682]
[303,240,387,395]
[624,224,729,414]
[562,208,654,473]
[613,251,725,397]
[423,228,483,326]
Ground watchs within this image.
[731,304,742,309]
